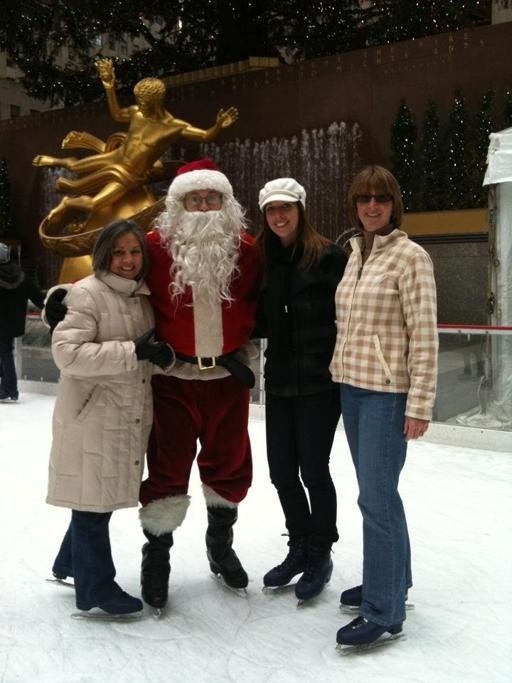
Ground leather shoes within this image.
[339,585,361,607]
[77,583,143,615]
[337,618,403,647]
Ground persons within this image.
[325,164,441,652]
[252,176,352,604]
[0,241,25,407]
[27,60,242,232]
[40,161,260,617]
[51,219,142,622]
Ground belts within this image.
[176,354,254,393]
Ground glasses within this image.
[183,193,222,205]
[355,192,391,203]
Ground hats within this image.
[258,178,307,211]
[169,158,234,210]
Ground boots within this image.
[205,505,249,589]
[295,535,334,598]
[262,528,305,587]
[139,532,173,607]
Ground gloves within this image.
[134,326,173,366]
[45,289,67,330]
